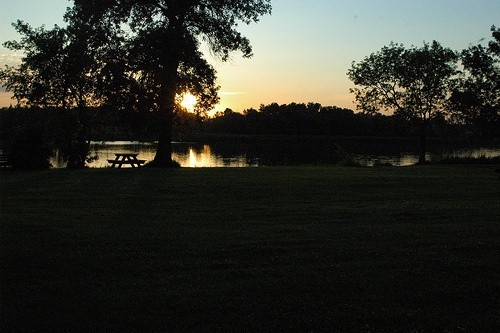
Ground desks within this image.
[108,154,147,168]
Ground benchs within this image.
[108,160,146,168]
[0,153,15,168]
